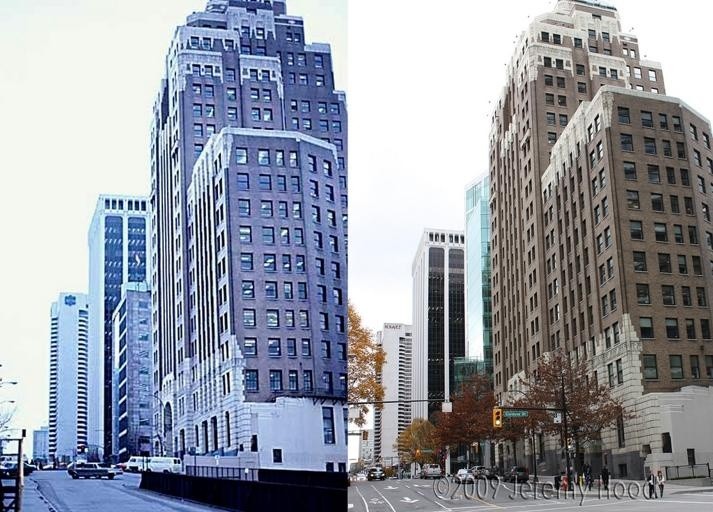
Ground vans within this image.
[418,463,443,480]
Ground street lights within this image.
[0,425,9,432]
[0,380,19,386]
[2,434,12,438]
[498,385,540,484]
[0,400,16,404]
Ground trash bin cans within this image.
[555,467,575,490]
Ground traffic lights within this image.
[415,448,420,456]
[492,408,503,428]
[362,432,368,440]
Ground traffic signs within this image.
[503,411,528,418]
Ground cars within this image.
[350,474,367,482]
[501,465,530,482]
[365,466,385,481]
[478,468,494,480]
[470,464,485,478]
[454,468,474,485]
[0,454,186,481]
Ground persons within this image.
[553,462,612,491]
[657,470,665,497]
[647,470,655,499]
